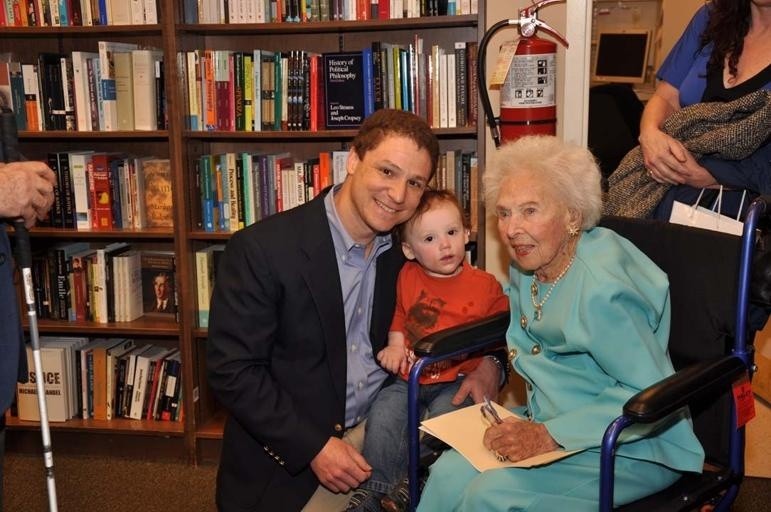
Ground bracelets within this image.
[488,356,502,368]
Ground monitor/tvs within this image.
[591,26,652,84]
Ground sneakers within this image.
[347,472,421,512]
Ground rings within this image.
[495,450,510,461]
[647,169,654,177]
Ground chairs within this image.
[407,198,770,510]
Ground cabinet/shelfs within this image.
[1,3,188,455]
[171,2,502,471]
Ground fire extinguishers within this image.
[477,0,568,150]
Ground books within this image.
[197,148,351,231]
[39,151,173,229]
[0,0,160,27]
[34,240,179,324]
[186,0,477,24]
[188,35,478,131]
[17,337,184,421]
[427,149,479,265]
[196,245,223,327]
[0,41,169,131]
[417,399,588,473]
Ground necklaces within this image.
[529,256,577,322]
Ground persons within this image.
[638,1,771,512]
[341,186,513,512]
[203,107,515,512]
[406,127,711,512]
[0,108,57,417]
